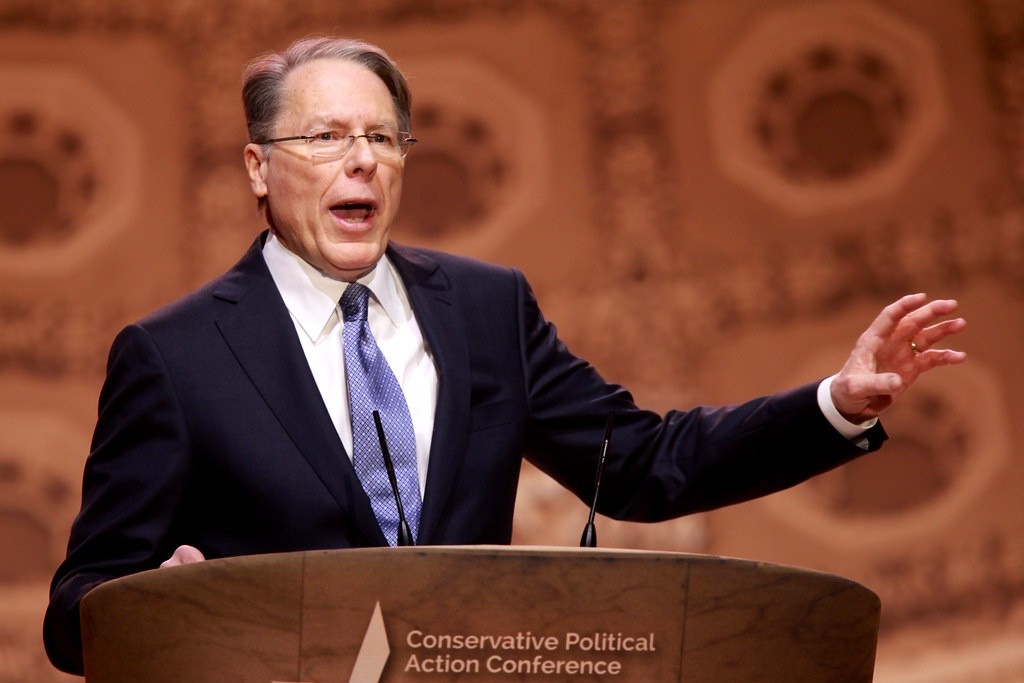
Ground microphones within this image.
[577,407,616,546]
[372,410,414,546]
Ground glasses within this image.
[253,125,417,160]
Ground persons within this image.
[42,38,967,676]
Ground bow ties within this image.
[339,283,421,547]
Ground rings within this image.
[910,340,922,354]
